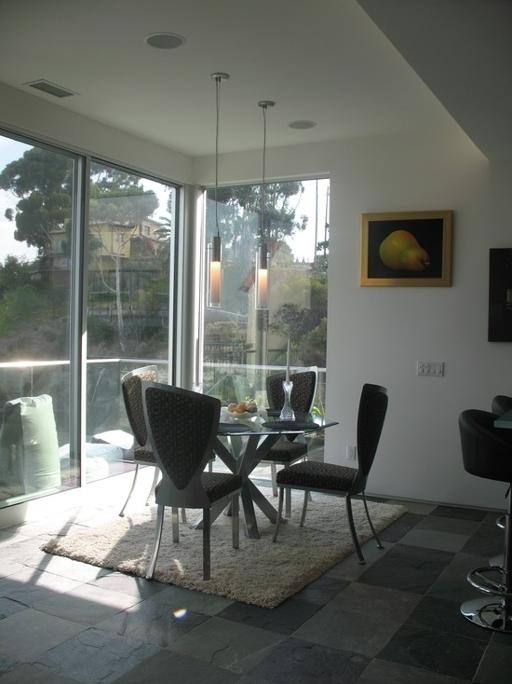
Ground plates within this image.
[225,409,259,418]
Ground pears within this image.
[379,230,431,272]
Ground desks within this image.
[192,415,340,552]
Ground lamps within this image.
[207,71,275,311]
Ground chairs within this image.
[226,364,320,518]
[272,383,387,567]
[119,365,195,524]
[135,381,247,580]
[458,395,512,634]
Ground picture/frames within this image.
[359,209,453,288]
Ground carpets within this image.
[38,484,409,611]
